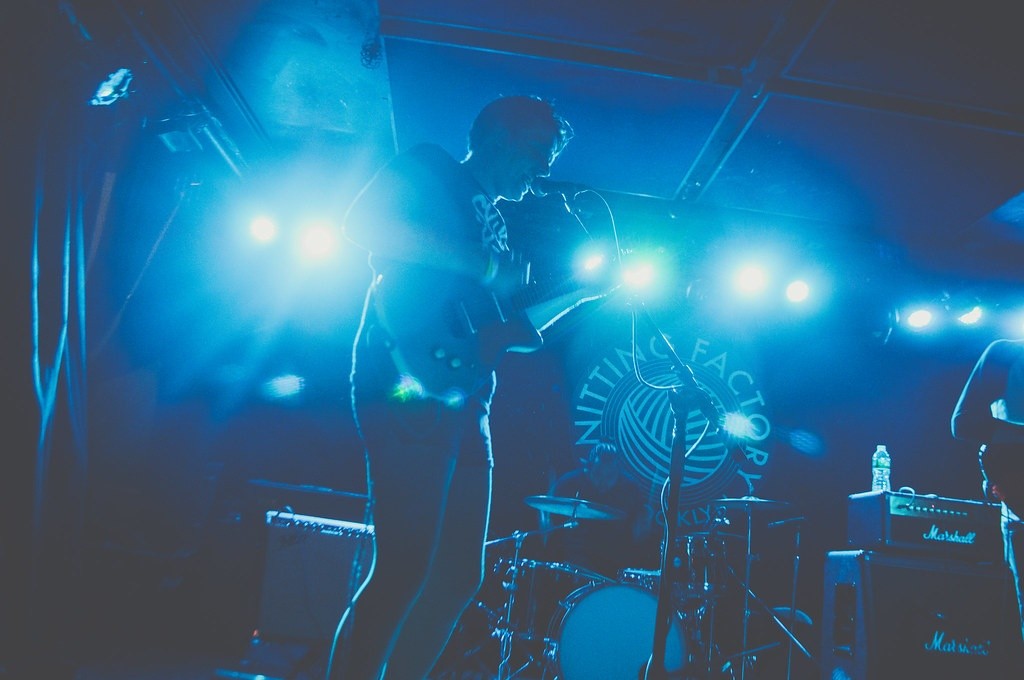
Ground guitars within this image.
[370,250,639,402]
[978,442,1024,523]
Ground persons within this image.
[325,95,625,680]
[948,335,1024,638]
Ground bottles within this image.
[872,445,891,492]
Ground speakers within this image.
[255,511,372,643]
[823,548,1024,680]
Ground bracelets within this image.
[478,251,499,288]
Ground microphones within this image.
[530,177,594,198]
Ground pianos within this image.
[234,477,374,512]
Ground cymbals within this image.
[717,494,792,513]
[522,492,630,524]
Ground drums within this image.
[493,555,543,594]
[538,559,614,605]
[619,566,700,611]
[669,530,749,596]
[540,581,689,679]
